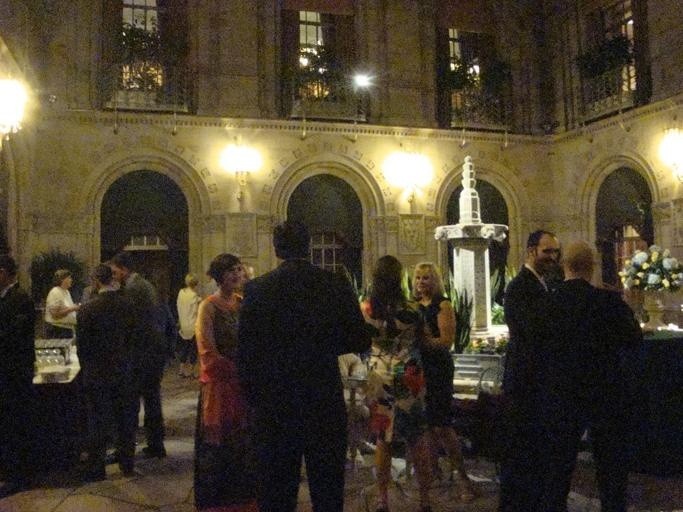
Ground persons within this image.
[238,221,373,512]
[76,264,144,481]
[539,240,644,512]
[499,230,560,512]
[192,253,253,512]
[109,254,166,459]
[412,262,476,503]
[359,255,432,512]
[177,274,201,378]
[0,257,35,499]
[40,270,80,339]
